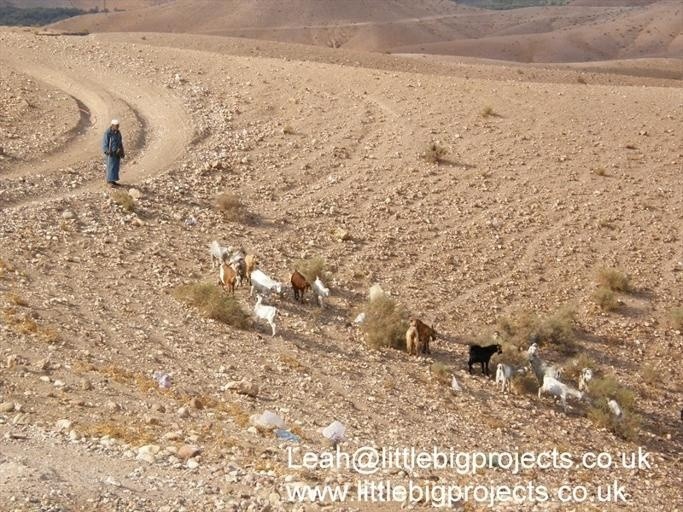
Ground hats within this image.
[110,119,119,125]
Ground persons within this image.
[102,119,124,188]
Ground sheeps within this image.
[494,363,513,393]
[527,343,537,374]
[538,374,586,413]
[253,292,284,339]
[291,269,312,303]
[312,276,330,311]
[404,319,438,354]
[247,270,285,299]
[467,341,504,376]
[207,240,259,299]
